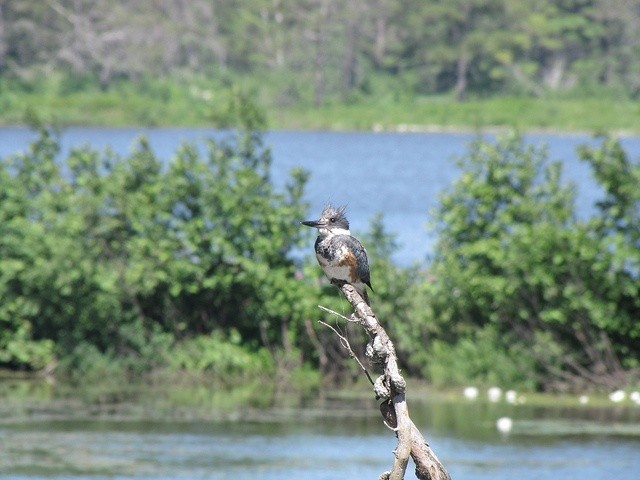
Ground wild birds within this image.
[299,207,375,309]
